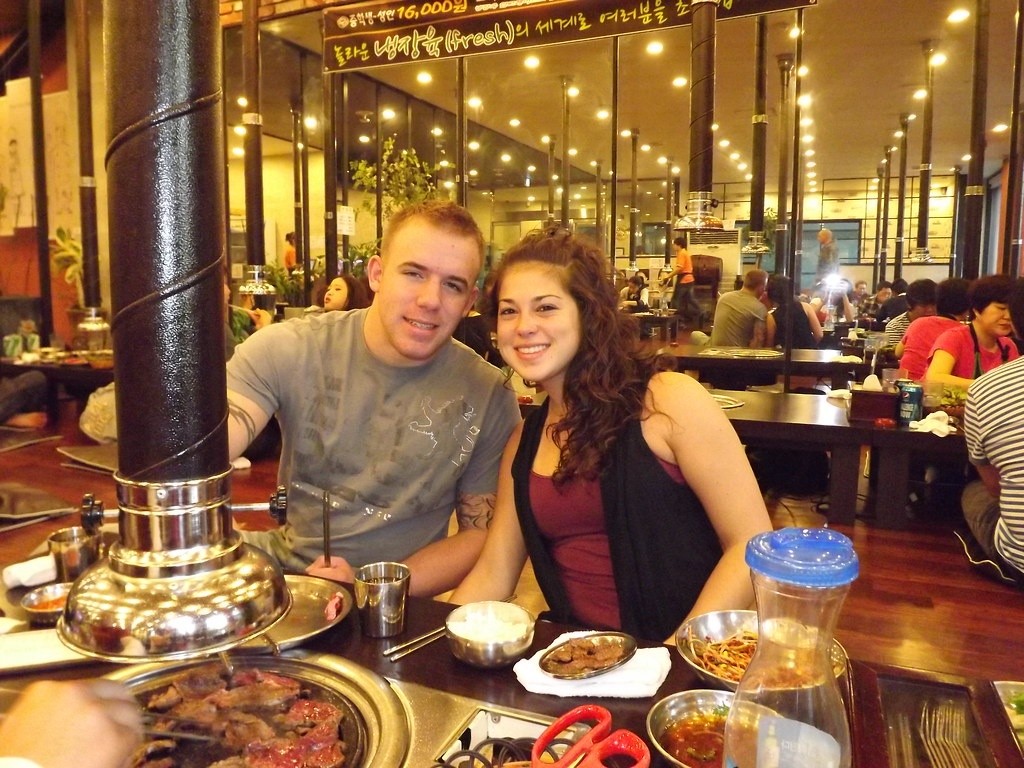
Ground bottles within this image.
[658,297,669,317]
[721,526,860,768]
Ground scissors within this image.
[531,705,650,768]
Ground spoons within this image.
[913,694,979,768]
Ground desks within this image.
[0,341,872,528]
[637,315,900,388]
[708,389,969,525]
[871,421,967,530]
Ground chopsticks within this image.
[321,490,332,568]
[0,687,216,741]
[383,594,519,662]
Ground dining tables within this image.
[1,572,1023,768]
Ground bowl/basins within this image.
[446,600,536,669]
[20,582,74,626]
[676,609,849,695]
[645,689,781,768]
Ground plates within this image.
[238,574,352,655]
[0,628,95,674]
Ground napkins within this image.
[910,411,949,437]
[513,631,673,697]
[827,388,850,399]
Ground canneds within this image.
[894,378,924,424]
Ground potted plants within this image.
[50,227,106,336]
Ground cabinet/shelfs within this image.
[231,215,277,267]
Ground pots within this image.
[97,648,409,768]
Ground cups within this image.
[914,380,944,420]
[46,526,101,583]
[353,560,411,637]
[864,339,881,361]
[882,368,908,389]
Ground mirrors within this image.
[224,25,608,308]
[642,219,862,274]
[221,0,1024,391]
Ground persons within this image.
[446,227,776,645]
[224,232,372,469]
[920,274,1024,588]
[226,201,522,599]
[454,273,505,369]
[0,371,51,428]
[614,237,823,390]
[797,278,972,383]
[0,678,145,768]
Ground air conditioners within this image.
[687,227,745,318]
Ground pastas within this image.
[685,626,825,688]
